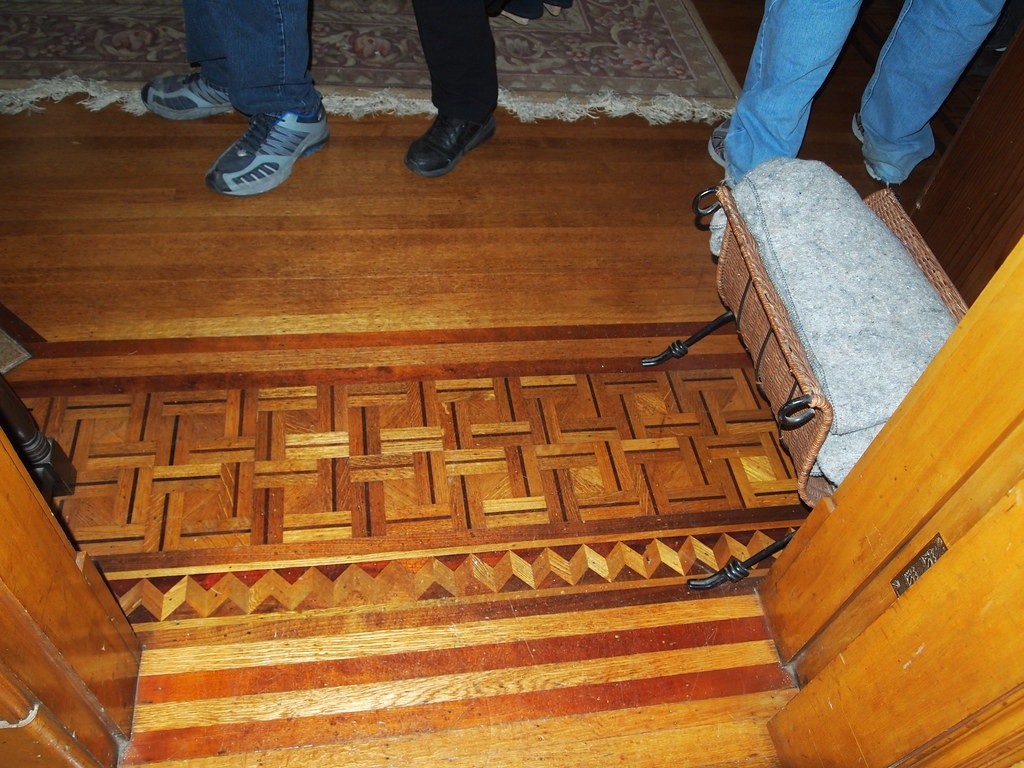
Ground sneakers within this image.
[207,102,330,195]
[709,119,734,191]
[852,111,881,182]
[141,74,232,119]
[406,114,500,176]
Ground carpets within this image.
[0,0,744,127]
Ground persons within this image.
[707,1,1007,190]
[494,0,573,26]
[141,0,330,195]
[404,1,501,178]
